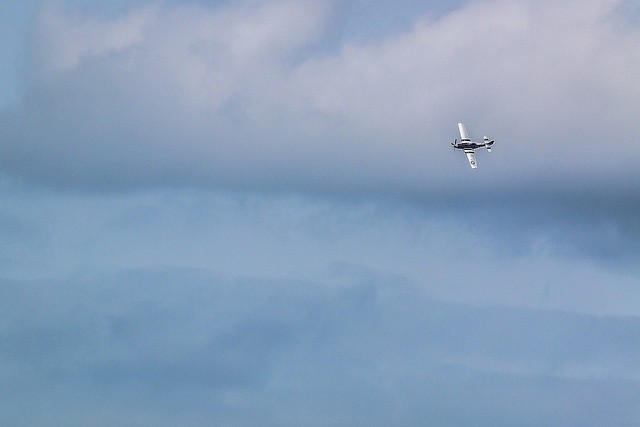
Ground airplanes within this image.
[451,123,495,169]
